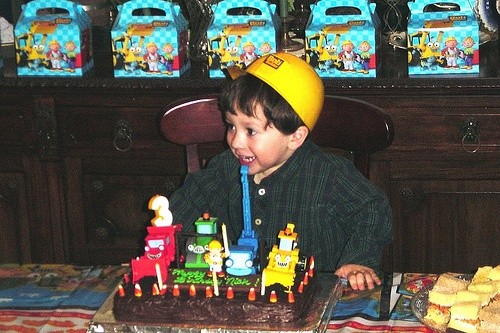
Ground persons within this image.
[164,52,392,291]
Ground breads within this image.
[424,264,500,333]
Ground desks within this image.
[0,263,437,333]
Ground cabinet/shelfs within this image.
[0,55,500,272]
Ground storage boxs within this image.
[14,0,96,77]
[205,0,280,78]
[111,0,192,78]
[407,0,480,75]
[305,0,383,79]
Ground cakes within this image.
[113,212,316,323]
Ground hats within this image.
[245,52,325,132]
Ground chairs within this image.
[158,94,393,178]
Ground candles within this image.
[222,223,229,256]
[212,268,219,296]
[261,272,265,296]
[156,264,163,289]
